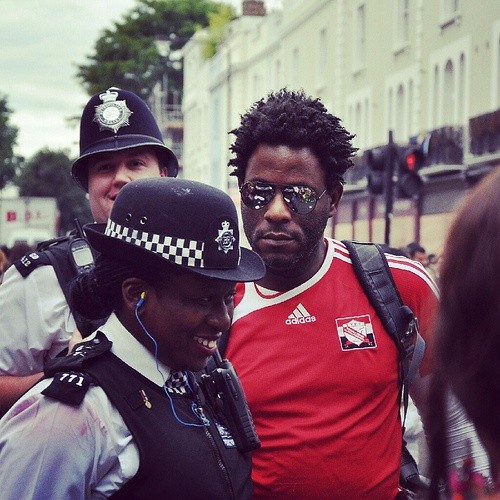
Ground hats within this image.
[82,177,266,282]
[71,87,179,194]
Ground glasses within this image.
[239,180,330,215]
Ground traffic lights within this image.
[397,144,424,200]
[364,148,386,195]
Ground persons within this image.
[0,87,180,415]
[424,166,500,500]
[406,241,426,264]
[0,176,267,500]
[217,87,489,500]
[0,241,40,274]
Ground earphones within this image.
[136,291,145,308]
